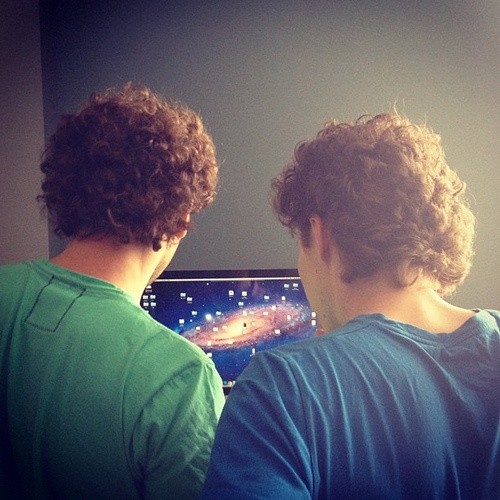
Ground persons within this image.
[0,81,226,500]
[202,98,500,500]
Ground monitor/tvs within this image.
[140,269,319,395]
